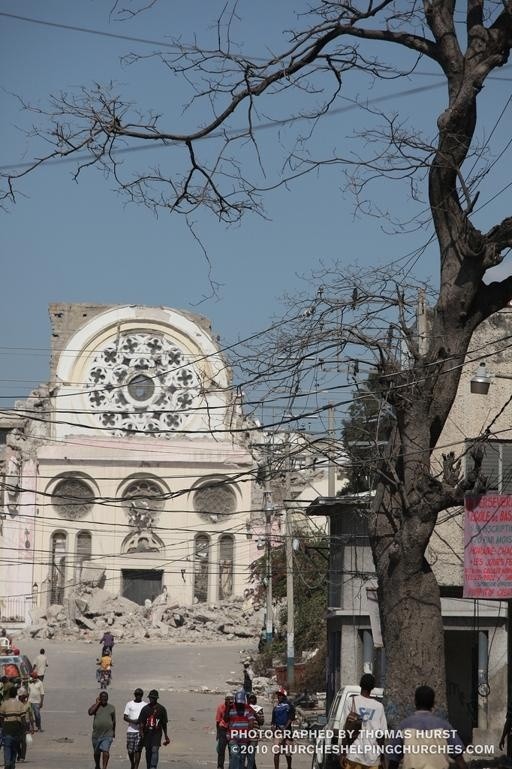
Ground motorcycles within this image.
[95,656,114,689]
[102,635,116,657]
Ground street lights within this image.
[257,539,272,579]
[470,363,512,395]
[276,508,321,534]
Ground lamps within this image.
[470,362,491,383]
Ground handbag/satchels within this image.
[342,712,362,745]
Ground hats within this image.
[147,690,159,698]
[235,692,246,704]
[277,688,287,696]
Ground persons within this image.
[99,650,112,682]
[88,691,116,769]
[124,688,148,769]
[387,685,465,769]
[270,688,295,769]
[0,628,49,769]
[100,631,115,655]
[499,703,511,768]
[215,662,265,769]
[339,674,390,769]
[138,689,170,769]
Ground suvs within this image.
[313,686,385,769]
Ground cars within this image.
[0,635,37,683]
